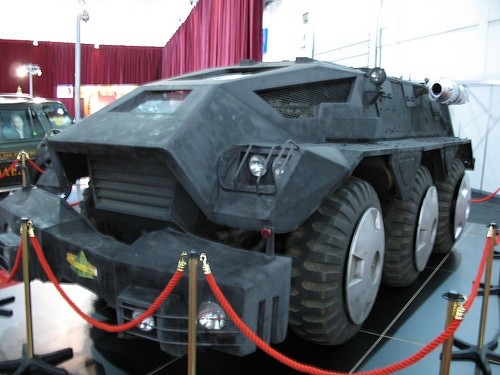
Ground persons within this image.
[5,113,34,139]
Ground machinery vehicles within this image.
[1,57,477,375]
